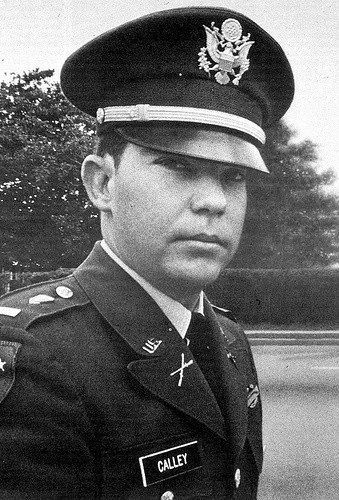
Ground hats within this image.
[59,5,295,175]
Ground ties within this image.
[187,313,227,420]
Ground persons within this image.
[0,5,297,500]
